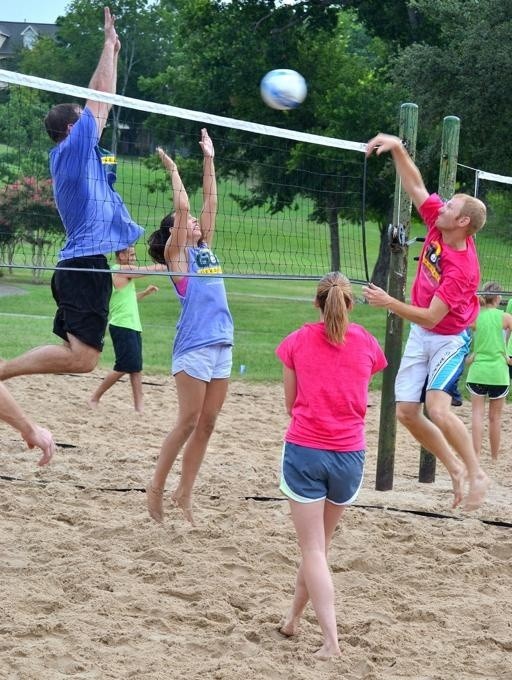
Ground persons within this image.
[0,380,57,466]
[148,127,235,530]
[274,269,388,661]
[0,5,145,382]
[465,281,512,467]
[362,131,490,513]
[504,298,512,347]
[85,243,168,411]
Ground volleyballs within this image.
[259,68,308,111]
